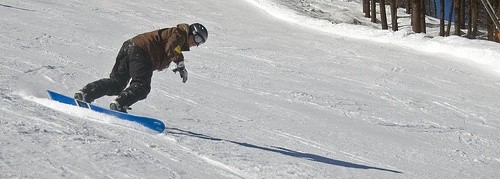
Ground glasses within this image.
[193,30,205,47]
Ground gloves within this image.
[172,61,188,83]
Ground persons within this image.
[74,23,208,114]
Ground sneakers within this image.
[109,98,132,114]
[74,87,94,104]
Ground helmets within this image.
[192,23,208,42]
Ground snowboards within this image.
[46,90,165,133]
